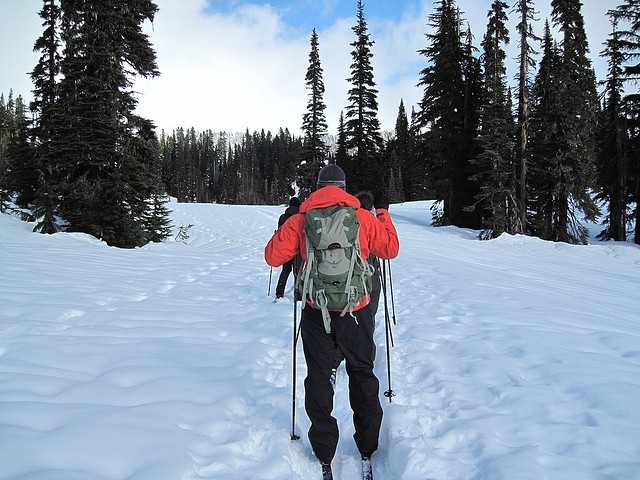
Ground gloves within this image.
[373,194,389,211]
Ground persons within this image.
[274,196,304,301]
[263,162,401,464]
[329,191,382,386]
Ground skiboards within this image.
[273,285,294,304]
[322,368,372,479]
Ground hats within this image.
[316,164,346,191]
[289,198,301,208]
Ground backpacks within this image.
[294,204,375,310]
